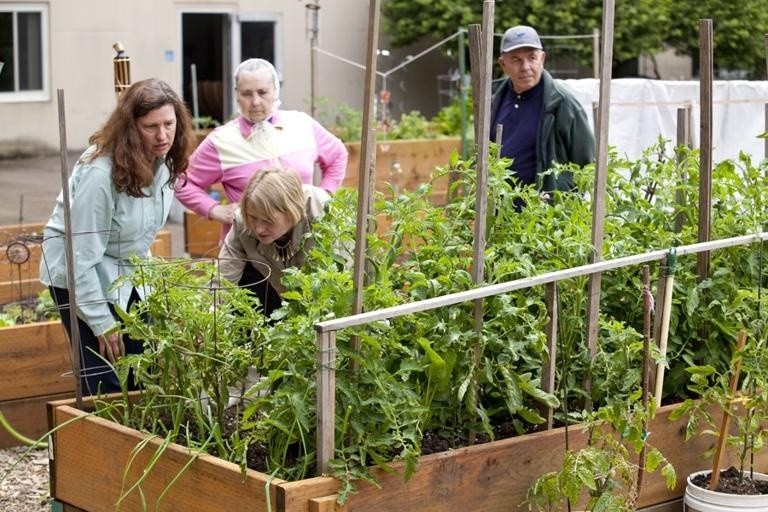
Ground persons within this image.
[490,25,596,215]
[180,163,394,350]
[38,77,196,398]
[172,55,350,252]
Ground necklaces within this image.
[271,239,295,264]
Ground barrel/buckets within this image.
[683,469,768,512]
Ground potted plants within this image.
[0,137,768,511]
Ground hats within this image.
[498,25,543,54]
